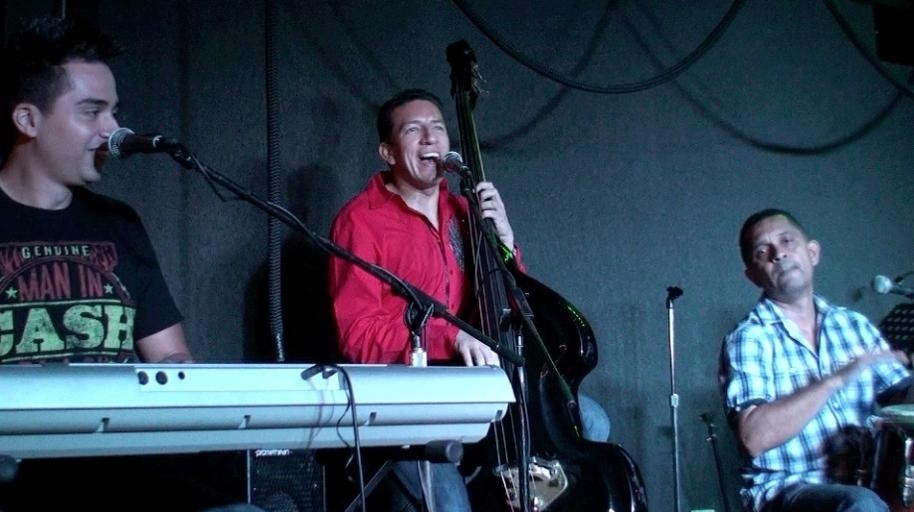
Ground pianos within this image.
[1,362,516,460]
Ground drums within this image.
[857,421,914,511]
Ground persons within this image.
[331,88,610,512]
[716,207,914,512]
[0,34,262,512]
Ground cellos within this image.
[445,38,650,510]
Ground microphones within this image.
[872,273,914,298]
[439,150,470,175]
[106,125,175,160]
